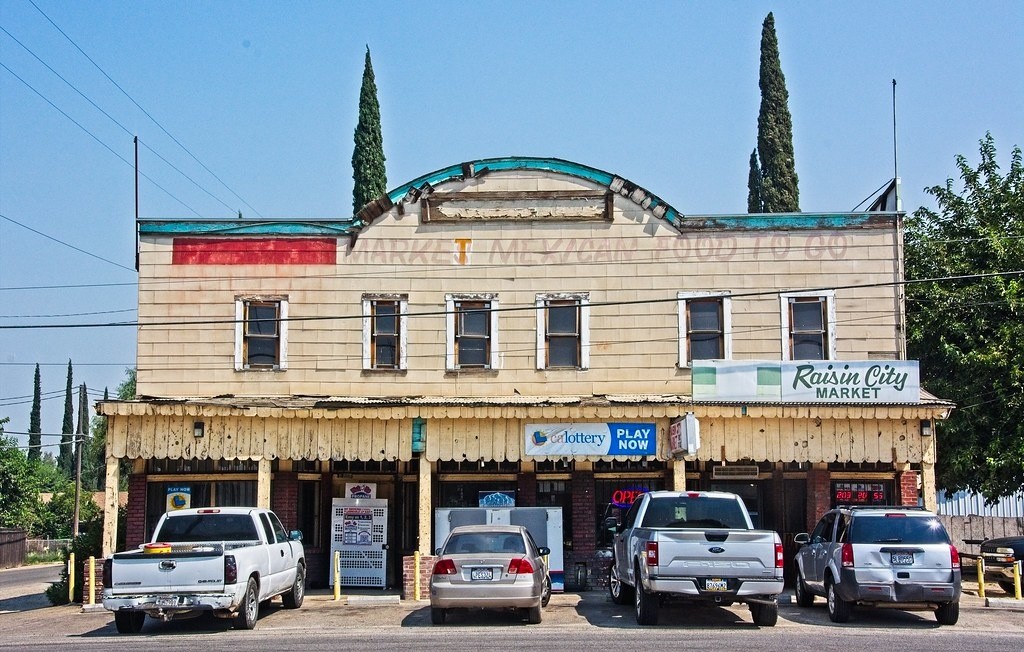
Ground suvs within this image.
[793,504,962,625]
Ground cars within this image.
[429,524,552,625]
[980,535,1024,597]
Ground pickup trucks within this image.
[609,490,784,626]
[103,506,306,632]
